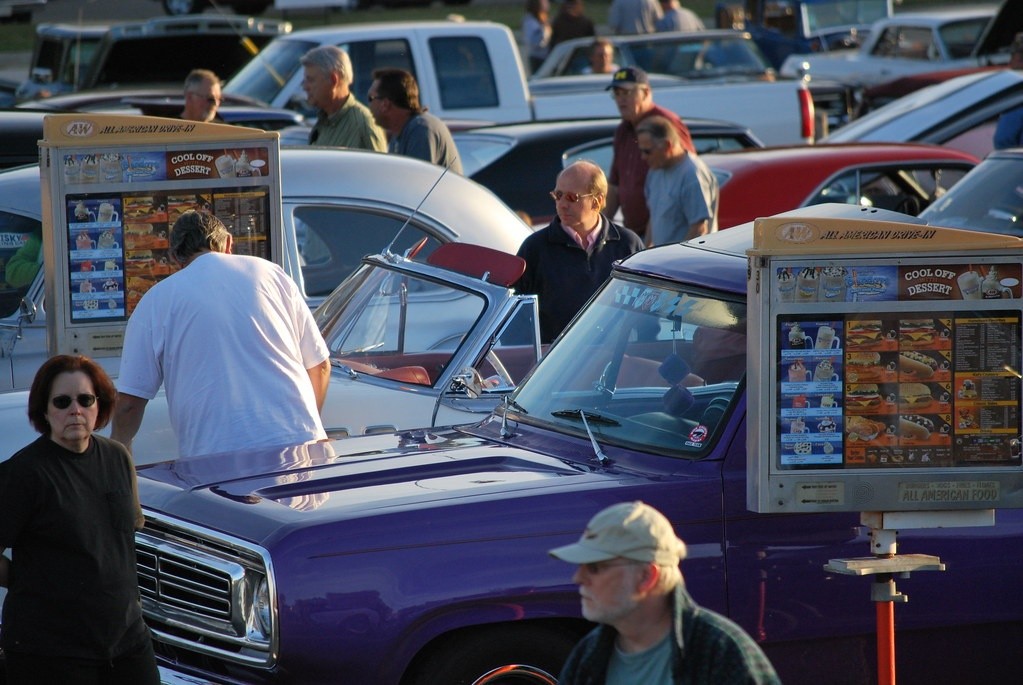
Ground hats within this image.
[548,500,679,565]
[605,67,648,90]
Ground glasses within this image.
[192,92,225,105]
[368,96,392,102]
[548,190,601,203]
[638,142,665,154]
[587,563,627,574]
[48,394,100,409]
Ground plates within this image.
[1000,278,1019,287]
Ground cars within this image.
[0,1,1022,616]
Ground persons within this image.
[500,161,661,346]
[367,69,464,175]
[0,355,161,685]
[110,210,332,456]
[300,45,390,153]
[179,70,223,122]
[548,501,784,685]
[635,115,719,247]
[525,0,747,75]
[602,67,696,248]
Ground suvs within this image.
[135,202,1023,685]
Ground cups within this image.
[821,396,837,407]
[75,208,96,223]
[105,284,119,292]
[813,367,838,381]
[958,271,982,299]
[792,395,810,408]
[236,162,261,176]
[105,265,119,270]
[65,165,80,183]
[215,155,234,178]
[80,162,99,183]
[778,280,795,300]
[76,240,96,249]
[97,237,119,249]
[80,263,95,272]
[815,326,839,348]
[789,369,812,382]
[818,273,846,302]
[80,284,96,292]
[791,421,810,433]
[788,332,814,349]
[99,160,123,183]
[795,276,817,301]
[983,283,1014,299]
[97,203,118,222]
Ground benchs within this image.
[530,346,708,389]
